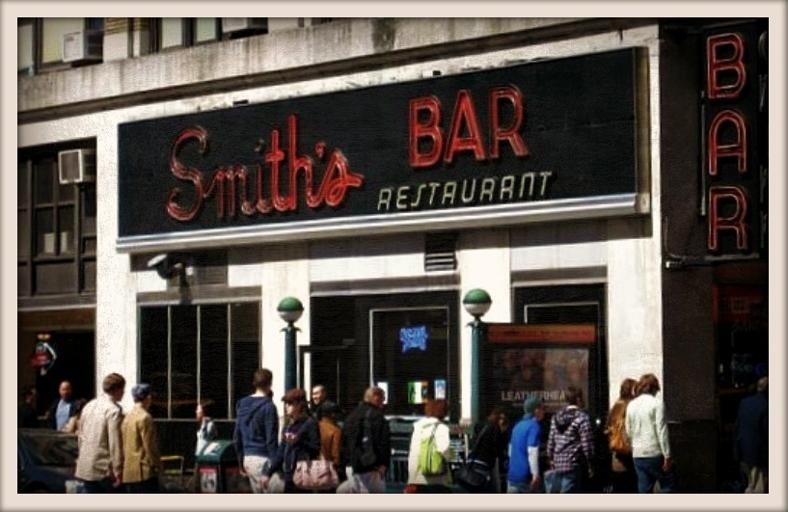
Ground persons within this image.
[601,373,678,492]
[75,373,126,492]
[466,407,511,492]
[505,395,546,492]
[233,369,391,492]
[408,399,456,492]
[546,385,595,492]
[19,381,88,434]
[194,399,218,458]
[739,375,768,493]
[120,384,166,492]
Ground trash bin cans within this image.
[195,439,254,494]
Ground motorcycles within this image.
[405,448,493,493]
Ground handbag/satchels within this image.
[609,417,632,454]
[294,460,339,489]
[456,465,484,488]
[349,406,381,474]
[420,437,445,475]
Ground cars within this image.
[17,425,83,493]
[145,368,224,415]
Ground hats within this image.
[132,384,156,398]
[523,398,542,419]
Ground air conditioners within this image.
[59,146,94,186]
[62,29,101,65]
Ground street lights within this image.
[275,292,307,426]
[462,286,495,445]
